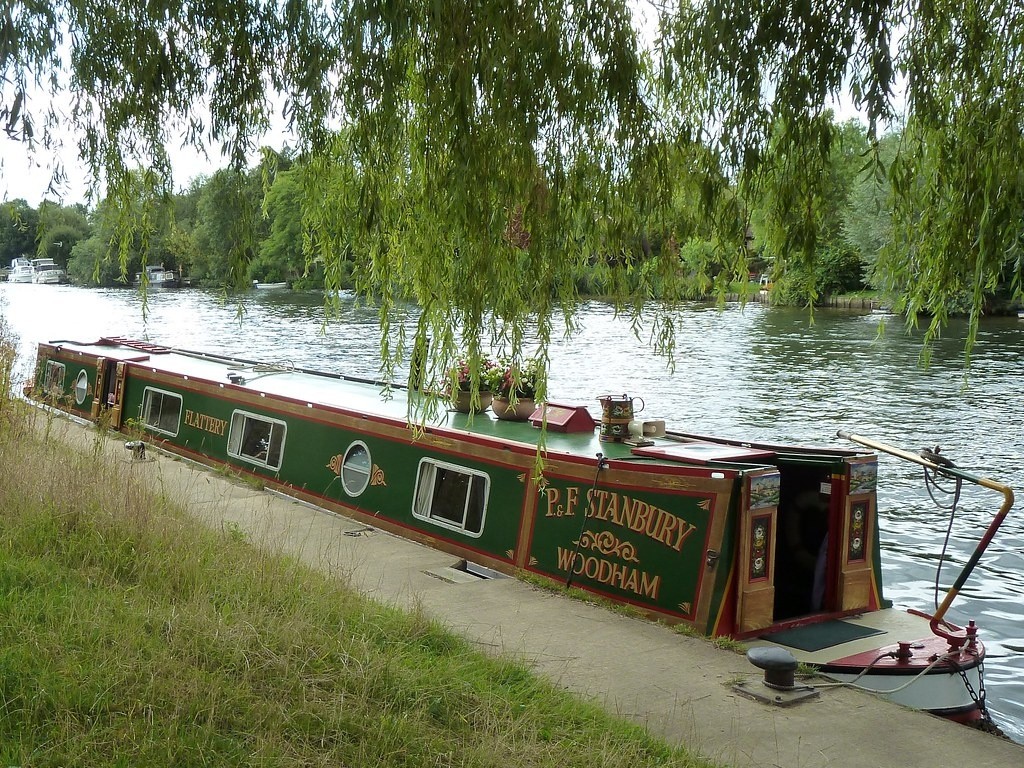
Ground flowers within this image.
[489,367,537,399]
[438,354,496,399]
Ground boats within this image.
[23,336,1015,724]
[329,288,357,299]
[31,258,65,284]
[133,262,183,288]
[6,253,32,283]
[256,282,286,289]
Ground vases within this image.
[451,391,491,412]
[492,397,535,419]
